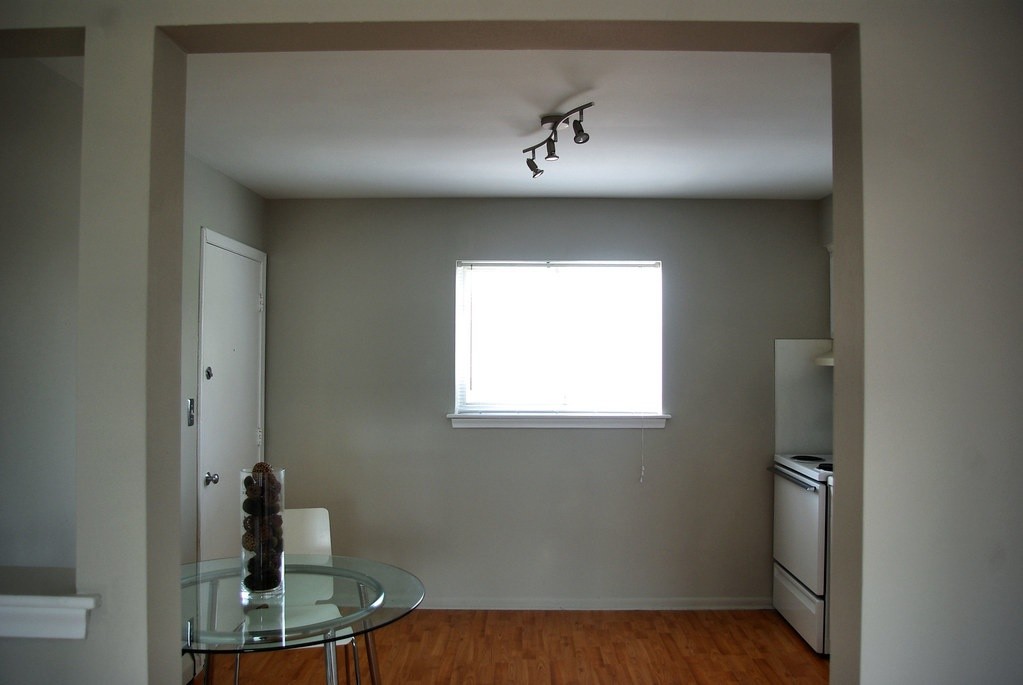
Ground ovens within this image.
[767,463,827,654]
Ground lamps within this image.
[522,101,595,181]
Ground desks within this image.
[180,553,427,685]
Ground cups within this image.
[239,466,286,599]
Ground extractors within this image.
[815,348,835,366]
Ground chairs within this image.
[234,509,362,685]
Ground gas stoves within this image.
[774,453,833,481]
